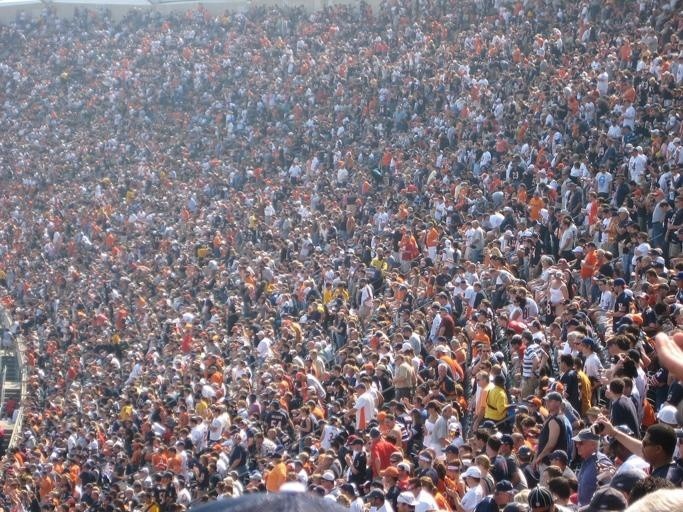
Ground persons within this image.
[0,0,683,512]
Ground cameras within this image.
[607,355,620,364]
[591,423,605,435]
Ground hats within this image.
[157,130,681,512]
